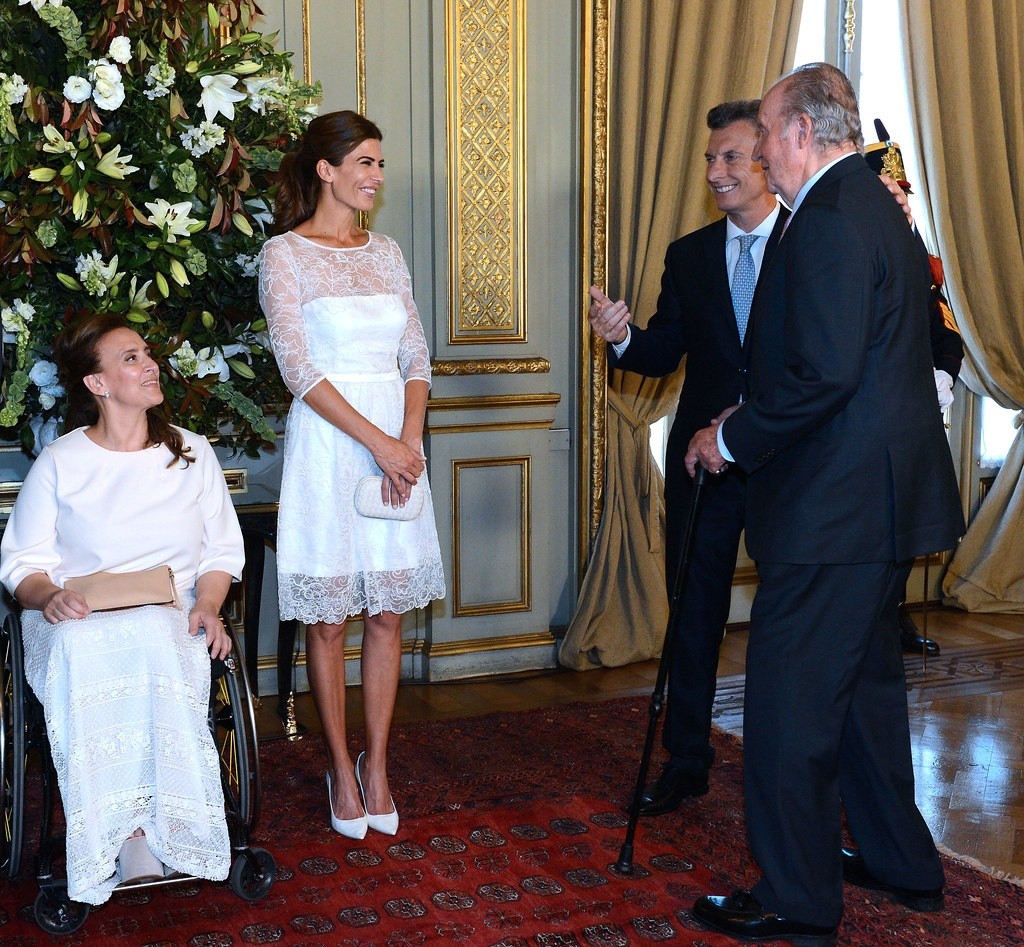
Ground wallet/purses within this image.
[353,474,424,522]
[62,565,181,614]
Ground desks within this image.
[0,483,303,743]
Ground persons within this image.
[588,100,931,817]
[0,311,246,905]
[258,110,446,839]
[682,63,966,947]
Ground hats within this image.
[863,117,913,193]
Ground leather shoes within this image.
[689,887,840,947]
[895,608,944,661]
[622,761,711,818]
[839,844,946,913]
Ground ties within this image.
[730,233,761,347]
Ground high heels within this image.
[354,748,399,838]
[318,769,373,841]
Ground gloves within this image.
[933,367,955,414]
